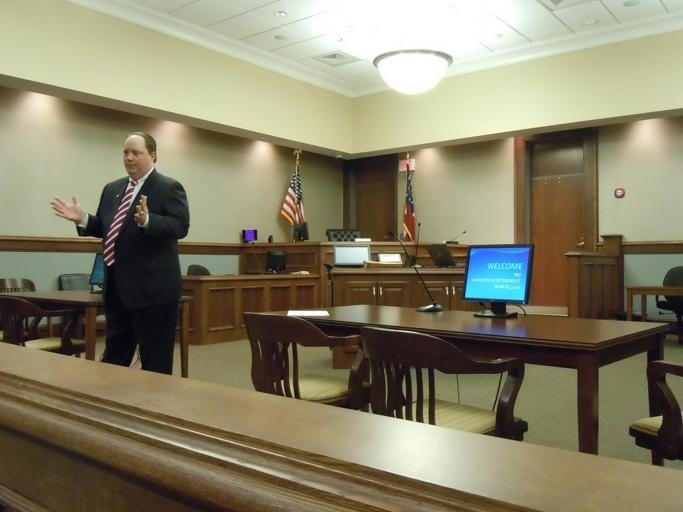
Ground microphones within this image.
[252,242,263,275]
[387,231,444,312]
[412,223,423,268]
[445,231,466,244]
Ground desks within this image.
[626,287,683,320]
[328,267,486,313]
[1,290,193,379]
[241,302,667,468]
[175,273,320,344]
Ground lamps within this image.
[373,50,453,97]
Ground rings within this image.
[140,211,144,215]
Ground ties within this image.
[103,179,137,267]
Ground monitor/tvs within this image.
[461,243,534,317]
[265,250,288,275]
[292,222,309,242]
[426,244,456,268]
[89,253,104,294]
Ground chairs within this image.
[0,265,114,363]
[630,349,682,469]
[362,326,530,447]
[657,266,683,344]
[241,309,370,412]
[326,229,362,242]
[187,264,211,276]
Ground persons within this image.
[52,131,190,377]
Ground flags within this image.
[280,152,305,243]
[402,157,416,242]
